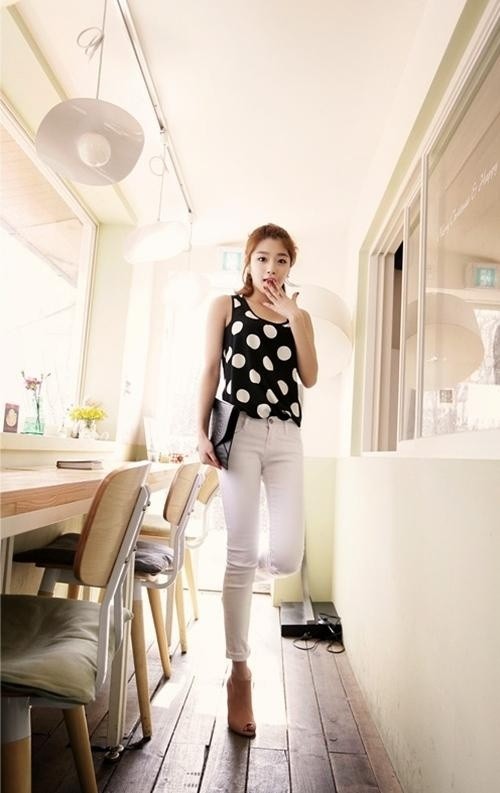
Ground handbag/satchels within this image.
[210,397,236,470]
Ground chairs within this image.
[4,462,152,791]
[27,462,203,741]
[94,463,212,655]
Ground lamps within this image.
[34,0,146,188]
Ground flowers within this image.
[21,368,52,430]
[65,398,108,421]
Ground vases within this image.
[21,397,47,433]
[78,419,99,440]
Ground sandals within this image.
[226,667,256,735]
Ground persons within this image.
[193,222,320,738]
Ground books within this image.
[56,459,104,471]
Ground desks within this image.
[1,453,176,595]
